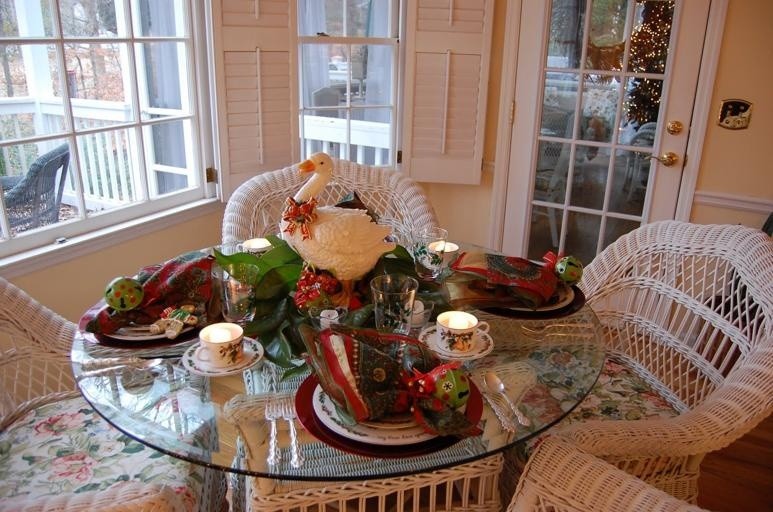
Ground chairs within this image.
[1,144,70,235]
[503,436,709,511]
[533,111,585,248]
[456,220,773,512]
[621,123,656,201]
[0,279,227,510]
[221,160,441,307]
[312,87,341,118]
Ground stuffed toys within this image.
[150,304,199,339]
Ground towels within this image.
[300,321,457,429]
[77,250,220,336]
[448,250,563,309]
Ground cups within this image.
[237,237,275,260]
[437,311,491,354]
[427,241,461,267]
[210,263,259,326]
[412,225,449,283]
[370,274,418,339]
[194,321,245,369]
[305,305,348,331]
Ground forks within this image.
[282,395,306,469]
[264,397,283,466]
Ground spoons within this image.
[486,372,531,428]
[469,374,516,433]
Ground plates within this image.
[181,336,264,377]
[79,301,203,351]
[418,325,494,363]
[506,284,578,312]
[294,371,483,460]
[311,382,467,447]
[482,281,586,321]
[96,301,204,341]
